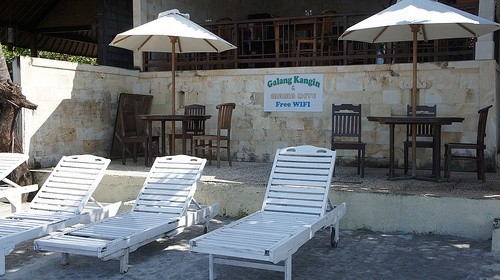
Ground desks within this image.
[133,115,211,166]
[275,16,322,67]
[366,116,464,182]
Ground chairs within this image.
[169,104,206,158]
[207,17,234,69]
[189,145,347,280]
[404,104,437,175]
[0,153,38,213]
[330,104,367,177]
[34,155,220,274]
[0,154,124,276]
[296,9,336,67]
[120,113,160,165]
[444,105,492,180]
[192,102,236,168]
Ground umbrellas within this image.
[109,9,238,155]
[338,0,500,177]
